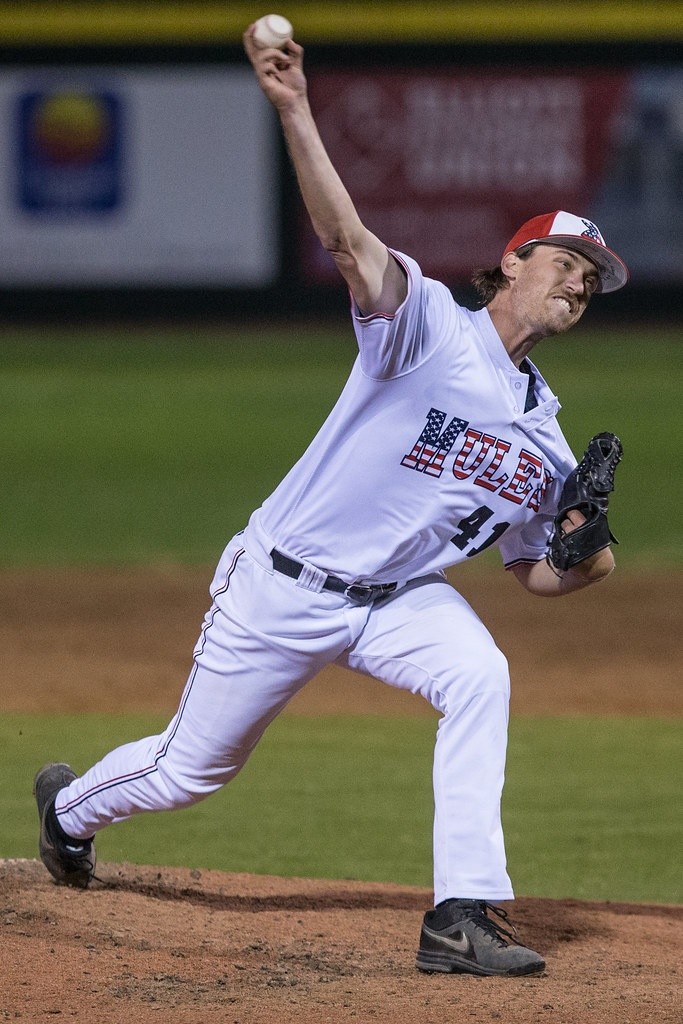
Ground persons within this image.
[31,25,629,977]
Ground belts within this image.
[270,548,409,605]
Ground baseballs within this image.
[252,13,293,51]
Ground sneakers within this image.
[415,898,546,977]
[34,763,96,888]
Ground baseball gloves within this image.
[547,429,625,569]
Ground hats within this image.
[502,210,629,293]
